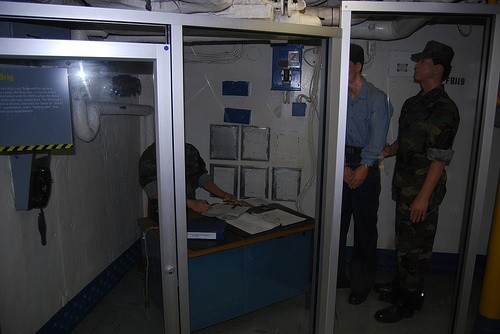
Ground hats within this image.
[412,40,455,63]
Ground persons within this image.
[138,141,237,219]
[337,43,394,305]
[373,40,460,323]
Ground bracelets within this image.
[360,164,371,168]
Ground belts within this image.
[344,147,361,154]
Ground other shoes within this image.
[348,278,374,305]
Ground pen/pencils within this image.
[205,199,210,205]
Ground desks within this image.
[138,196,315,332]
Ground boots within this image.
[373,276,400,292]
[374,292,418,322]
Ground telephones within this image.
[33,165,53,209]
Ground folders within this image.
[186,219,228,241]
